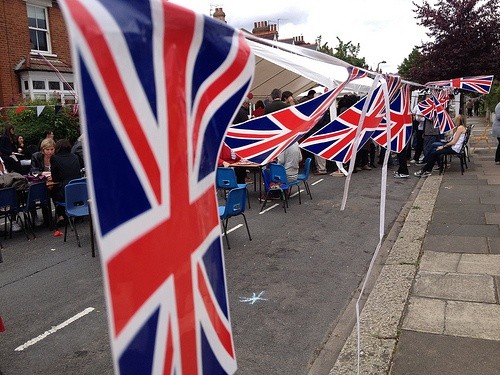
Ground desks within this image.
[229,162,261,192]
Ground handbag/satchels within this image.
[425,119,439,136]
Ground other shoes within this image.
[421,171,432,177]
[363,164,372,170]
[370,162,377,168]
[314,171,327,175]
[407,163,411,167]
[394,170,409,178]
[56,220,65,227]
[414,169,423,176]
[415,161,427,166]
[356,168,361,171]
[268,193,280,200]
[434,168,440,171]
[392,171,398,174]
[410,159,418,163]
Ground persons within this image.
[257,141,303,203]
[217,85,377,177]
[0,124,86,231]
[492,102,500,165]
[466,97,482,116]
[217,139,247,185]
[378,99,467,178]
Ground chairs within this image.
[438,123,492,175]
[0,176,52,264]
[259,158,311,213]
[55,176,88,247]
[216,165,252,250]
